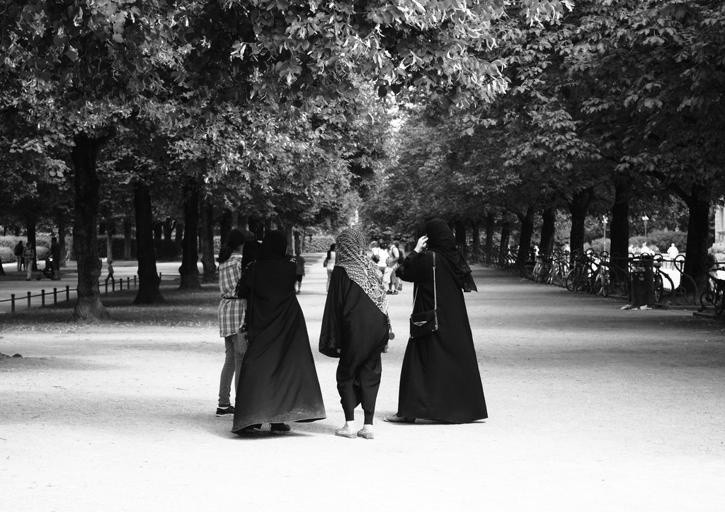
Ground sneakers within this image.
[216,405,235,417]
[383,415,418,422]
[269,423,291,433]
[357,428,374,439]
[334,427,357,438]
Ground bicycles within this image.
[469,240,724,314]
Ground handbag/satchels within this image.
[409,309,448,340]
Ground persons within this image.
[381,218,489,426]
[211,227,257,420]
[14,237,62,280]
[320,236,403,295]
[105,260,115,283]
[317,226,394,440]
[230,232,327,437]
[291,249,306,295]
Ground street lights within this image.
[641,214,649,236]
[602,215,608,255]
